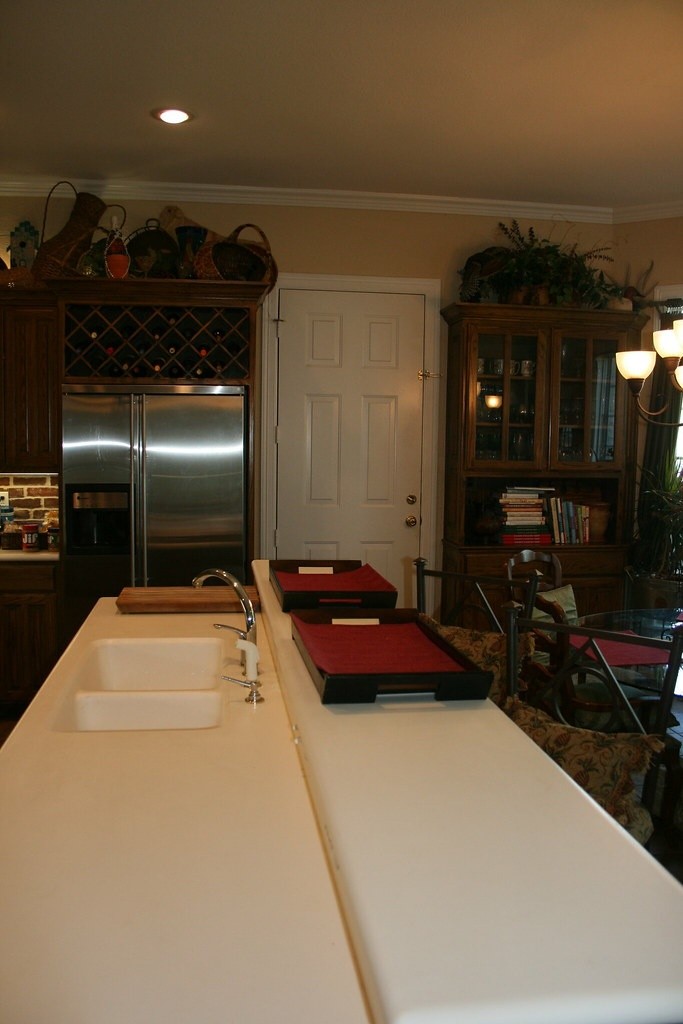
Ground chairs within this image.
[503,601,682,843]
[414,559,542,697]
[501,547,577,627]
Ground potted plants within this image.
[461,222,605,306]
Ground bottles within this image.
[76,314,240,379]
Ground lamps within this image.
[614,309,683,429]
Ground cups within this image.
[489,359,504,375]
[477,358,484,374]
[520,360,535,376]
[510,359,519,376]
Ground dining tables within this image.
[567,603,683,704]
[0,558,679,1024]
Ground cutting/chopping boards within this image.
[115,586,260,615]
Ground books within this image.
[499,487,590,546]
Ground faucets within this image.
[191,566,264,679]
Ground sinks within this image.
[54,635,225,731]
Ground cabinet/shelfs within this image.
[435,293,650,625]
[0,277,62,716]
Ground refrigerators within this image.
[59,383,247,657]
